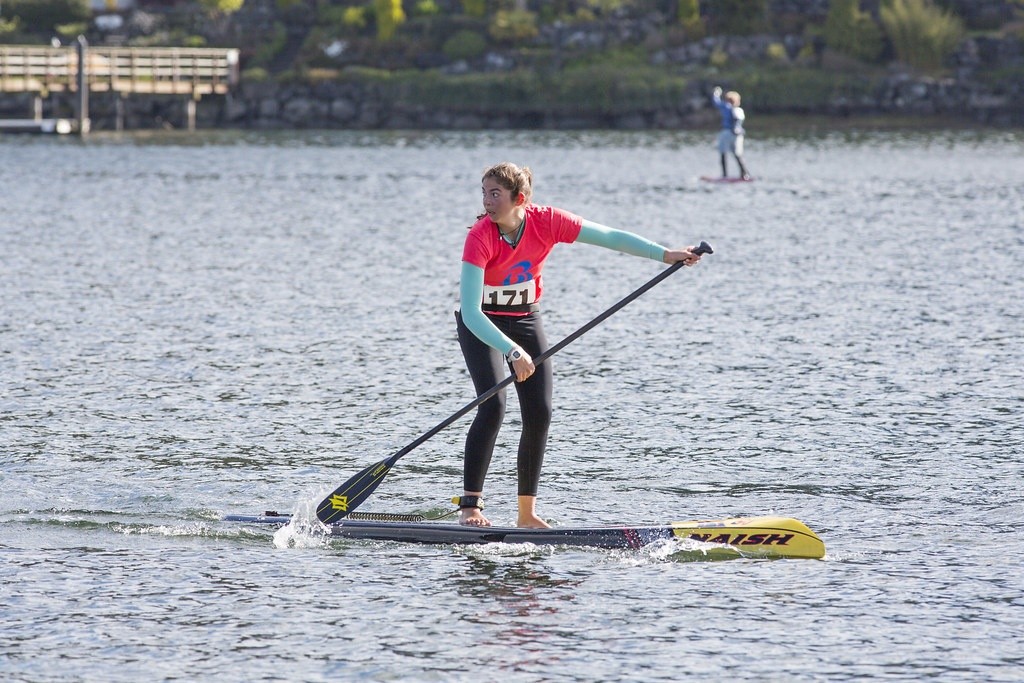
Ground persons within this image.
[455,162,700,528]
[712,86,749,179]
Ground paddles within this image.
[314,238,715,526]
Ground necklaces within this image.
[499,222,522,235]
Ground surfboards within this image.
[700,175,761,184]
[223,511,828,562]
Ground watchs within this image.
[508,348,524,362]
